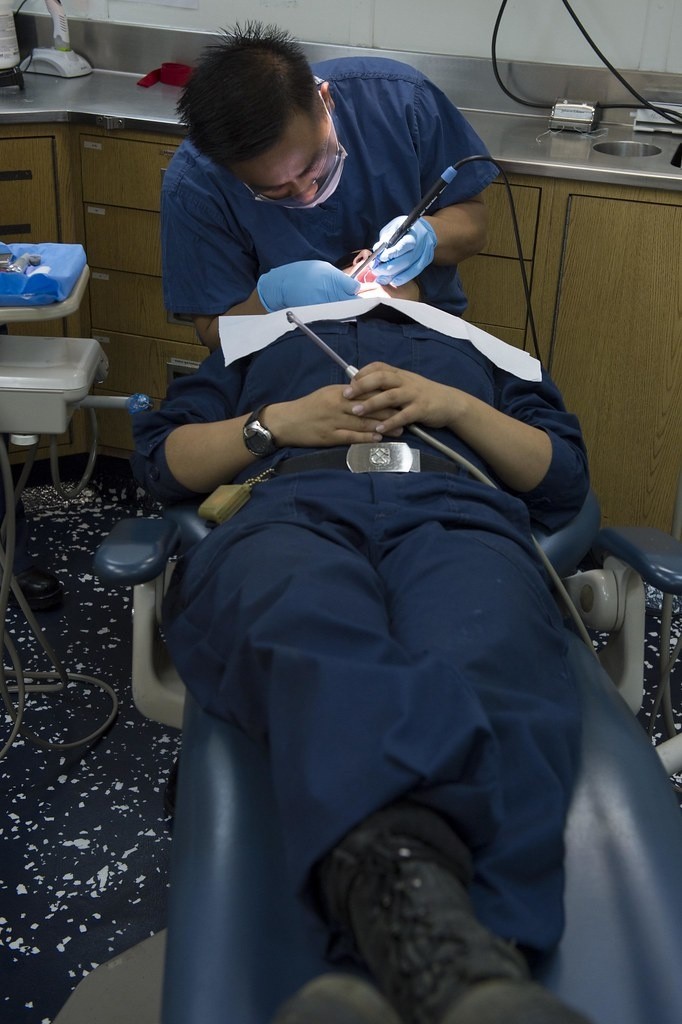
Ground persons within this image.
[124,245,597,1024]
[158,20,492,351]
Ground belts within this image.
[269,443,484,482]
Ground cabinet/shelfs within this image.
[0,123,682,547]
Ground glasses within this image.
[252,145,328,201]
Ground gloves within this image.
[373,215,437,287]
[258,260,363,313]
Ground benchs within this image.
[56,369,682,1024]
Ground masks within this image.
[244,90,348,208]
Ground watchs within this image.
[241,401,278,459]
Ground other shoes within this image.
[272,973,593,1024]
